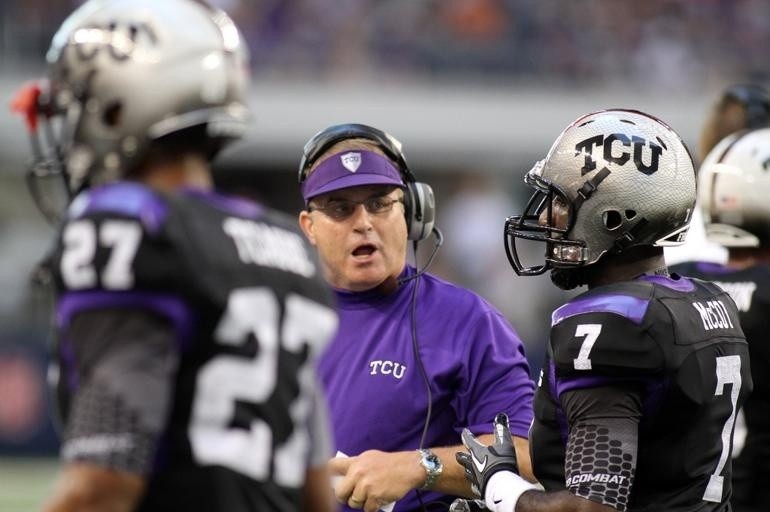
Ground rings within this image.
[351,493,362,504]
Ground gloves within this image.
[447,411,520,512]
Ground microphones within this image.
[381,229,444,296]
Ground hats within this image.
[298,147,410,200]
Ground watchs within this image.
[417,448,444,491]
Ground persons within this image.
[9,2,341,512]
[297,125,536,511]
[662,127,769,511]
[448,110,753,510]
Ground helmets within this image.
[525,106,698,270]
[44,0,253,187]
[698,127,770,249]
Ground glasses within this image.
[309,194,402,220]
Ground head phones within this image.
[296,121,437,243]
